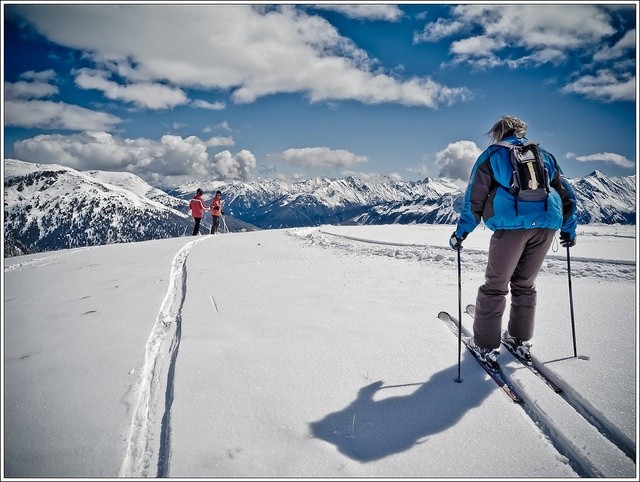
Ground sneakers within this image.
[503,330,531,359]
[467,337,500,363]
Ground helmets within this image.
[197,188,203,195]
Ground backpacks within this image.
[489,140,552,216]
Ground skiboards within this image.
[439,303,562,403]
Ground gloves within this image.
[221,210,224,214]
[449,232,465,250]
[214,206,220,210]
[559,232,577,248]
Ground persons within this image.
[449,116,578,362]
[210,189,224,235]
[190,189,211,236]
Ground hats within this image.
[216,191,221,194]
